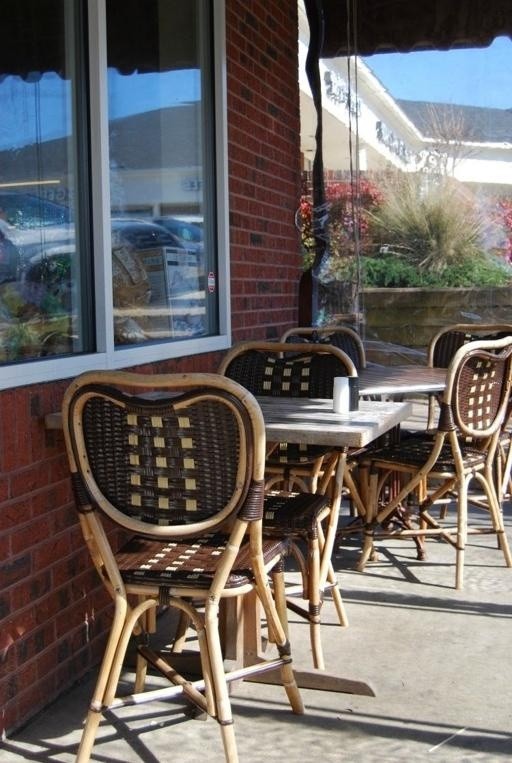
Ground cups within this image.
[348,376,359,411]
[332,376,350,412]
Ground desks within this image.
[47,388,413,673]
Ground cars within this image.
[0,188,206,318]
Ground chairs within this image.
[279,319,511,369]
[358,335,511,589]
[59,368,306,761]
[170,343,359,676]
